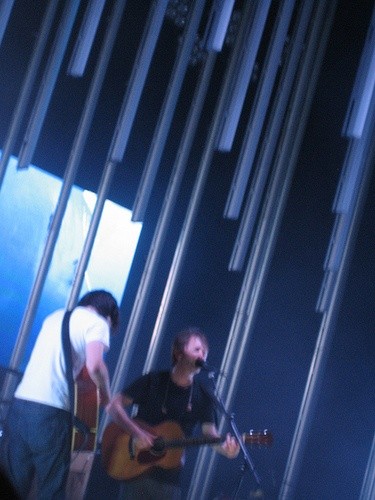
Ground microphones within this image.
[196,358,218,374]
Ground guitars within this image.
[101,417,273,481]
[68,361,100,454]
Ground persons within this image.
[105,327,246,500]
[5,290,119,500]
